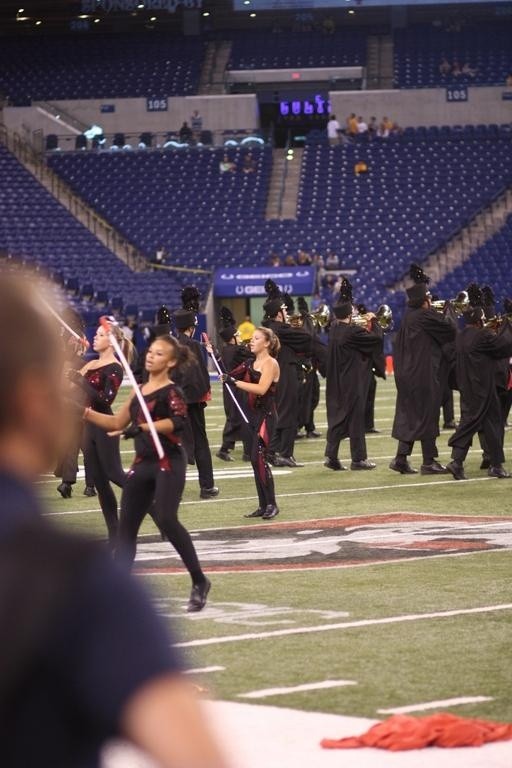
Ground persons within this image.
[326,114,342,148]
[178,121,195,143]
[70,316,170,549]
[438,51,478,82]
[0,256,231,767]
[346,111,404,141]
[0,262,512,500]
[352,159,368,178]
[114,333,213,614]
[218,325,282,518]
[190,108,203,135]
[268,247,344,269]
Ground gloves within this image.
[221,374,237,387]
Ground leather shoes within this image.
[187,577,211,612]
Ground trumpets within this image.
[285,304,330,327]
[430,291,470,318]
[483,311,512,328]
[350,304,394,330]
